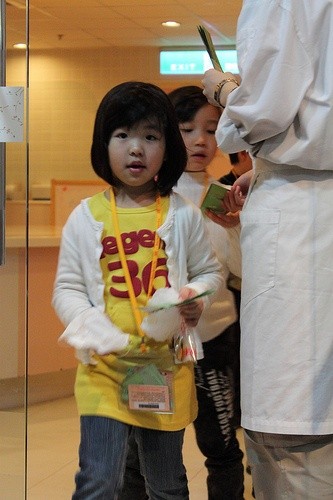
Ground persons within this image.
[159,85,243,500]
[212,0,333,500]
[50,81,230,500]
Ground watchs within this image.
[214,78,239,109]
